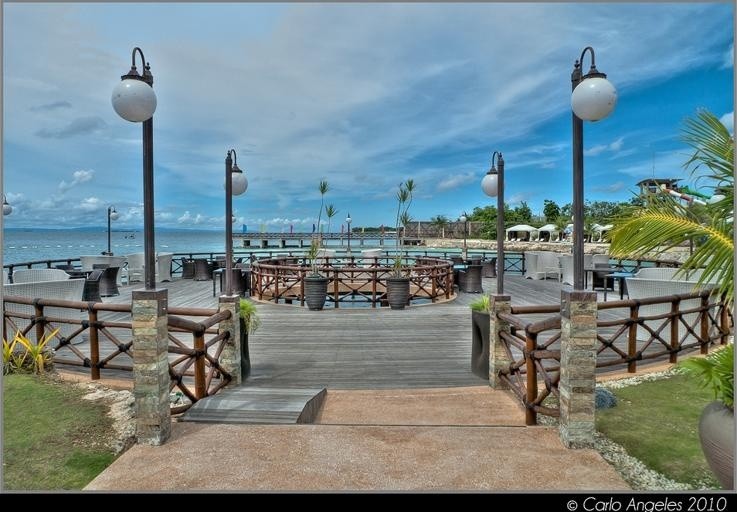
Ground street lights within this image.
[460,211,467,262]
[1,193,13,215]
[346,213,352,263]
[559,46,617,449]
[101,204,118,257]
[481,151,511,389]
[218,149,248,385]
[112,46,169,445]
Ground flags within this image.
[242,223,385,236]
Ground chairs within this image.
[450,254,497,293]
[2,250,252,351]
[523,250,728,348]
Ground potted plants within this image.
[668,341,735,490]
[469,293,494,379]
[235,299,262,382]
[386,175,415,311]
[302,178,339,312]
